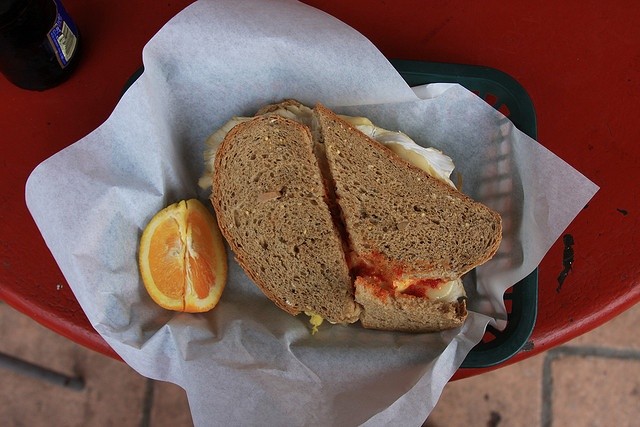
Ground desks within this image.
[1,0,639,382]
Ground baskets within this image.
[121,58,539,369]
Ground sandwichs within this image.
[197,97,503,335]
